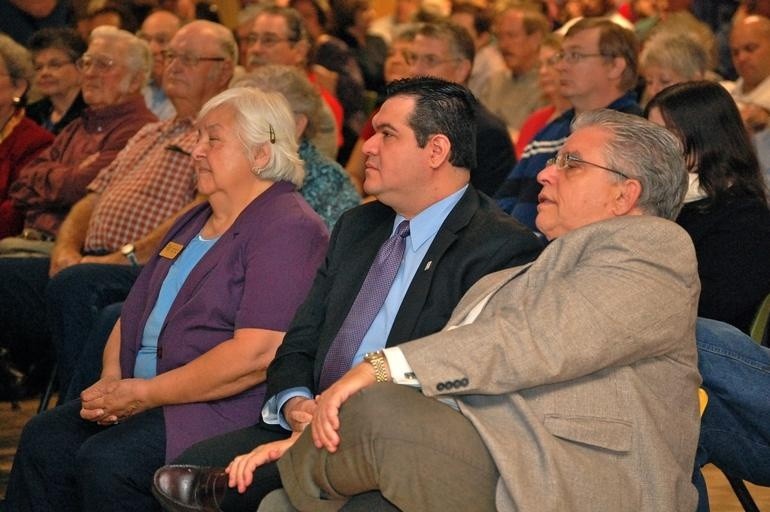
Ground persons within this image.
[716,14,769,134]
[25,27,87,135]
[135,9,181,120]
[1,84,329,511]
[402,22,474,87]
[467,9,549,143]
[515,35,571,164]
[1,20,238,403]
[1,24,160,257]
[634,13,722,113]
[691,318,769,512]
[246,6,344,165]
[167,78,549,465]
[549,1,715,45]
[644,77,769,335]
[1,32,54,238]
[494,18,650,246]
[1,1,139,40]
[245,8,344,161]
[149,109,701,511]
[227,66,361,241]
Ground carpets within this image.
[321,219,411,398]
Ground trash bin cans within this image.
[364,350,389,384]
[120,243,139,268]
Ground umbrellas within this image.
[151,462,252,512]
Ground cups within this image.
[403,52,465,68]
[76,56,139,75]
[164,48,227,66]
[32,58,74,71]
[553,50,616,64]
[247,30,305,48]
[544,151,637,180]
